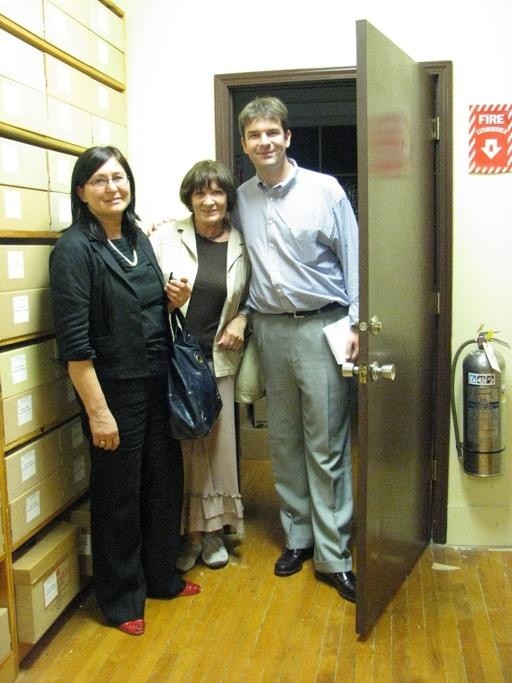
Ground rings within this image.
[182,292,188,298]
[178,297,184,303]
[100,441,105,445]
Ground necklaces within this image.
[195,226,225,240]
[104,236,139,268]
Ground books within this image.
[318,313,358,370]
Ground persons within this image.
[51,143,202,636]
[149,158,252,574]
[148,91,360,603]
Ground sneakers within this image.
[175,540,203,572]
[201,533,228,567]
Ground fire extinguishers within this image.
[452,327,511,479]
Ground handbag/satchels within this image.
[167,329,223,440]
[235,333,266,404]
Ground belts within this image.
[277,302,340,317]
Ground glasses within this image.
[87,175,130,188]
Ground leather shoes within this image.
[274,547,314,575]
[176,582,201,596]
[117,619,145,635]
[315,571,357,602]
